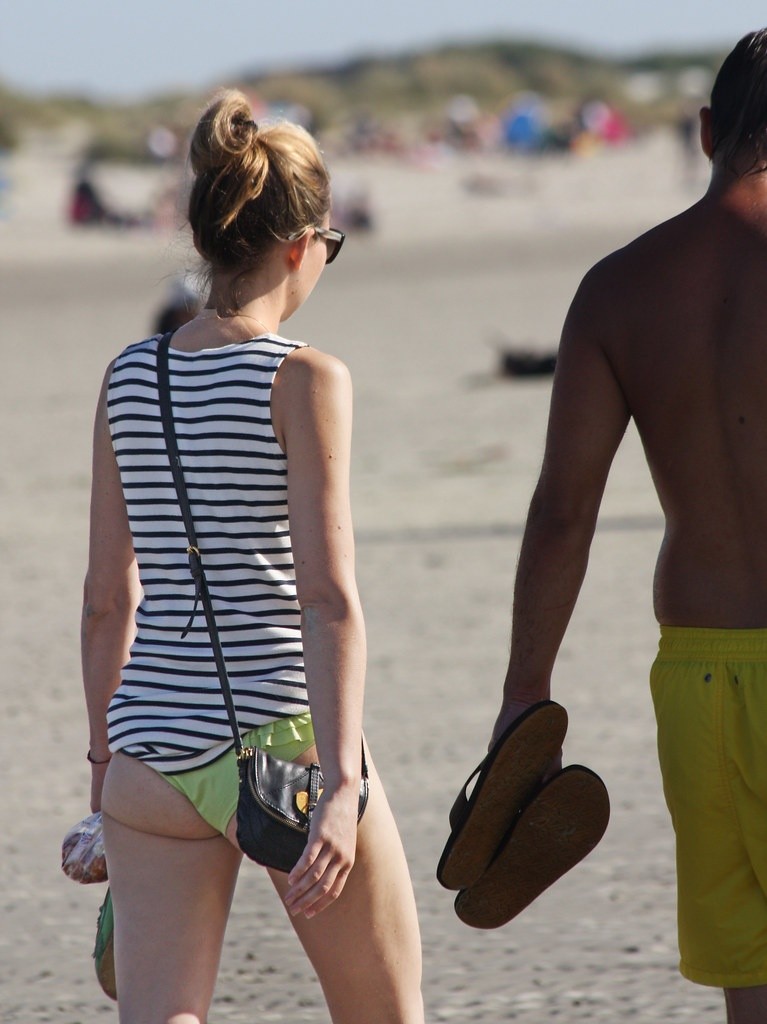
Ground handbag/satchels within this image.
[61,811,108,884]
[234,745,370,873]
[90,885,117,1001]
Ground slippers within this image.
[454,764,611,929]
[436,700,568,891]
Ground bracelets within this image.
[87,750,110,763]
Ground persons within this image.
[489,29,767,1024]
[80,86,427,1024]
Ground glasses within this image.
[287,228,345,265]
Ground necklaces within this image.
[194,314,271,332]
